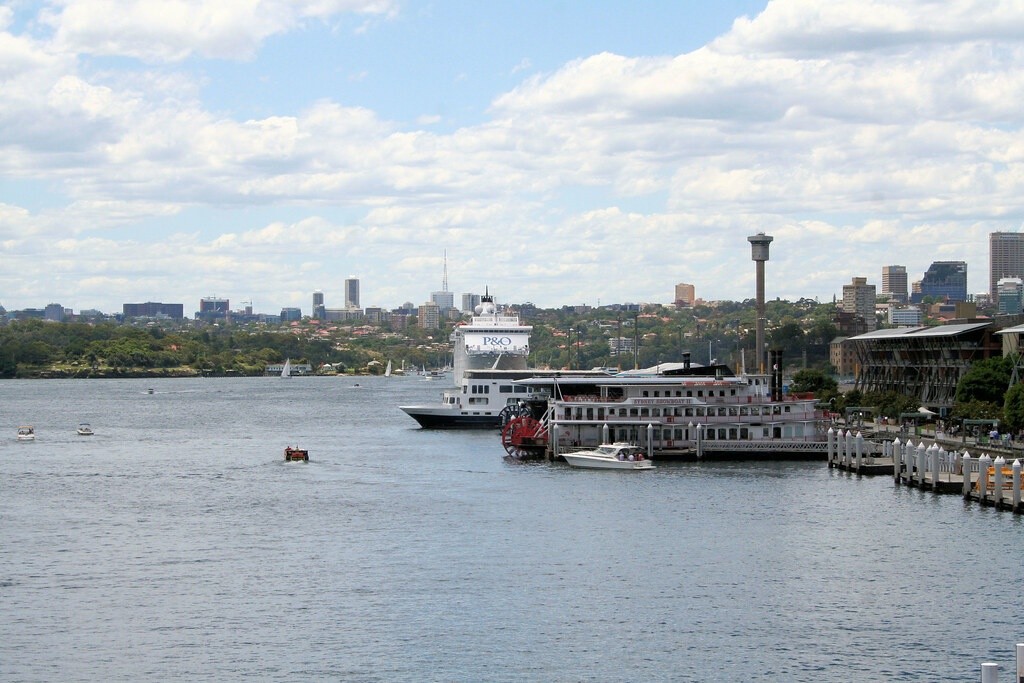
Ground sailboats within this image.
[422,364,427,376]
[384,359,392,378]
[280,357,292,379]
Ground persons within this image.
[990,428,999,447]
[619,452,644,461]
[1004,431,1015,450]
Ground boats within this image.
[417,374,446,381]
[514,374,889,458]
[76,422,94,435]
[17,426,34,440]
[398,368,614,430]
[559,442,655,470]
[148,388,153,394]
[283,446,310,462]
